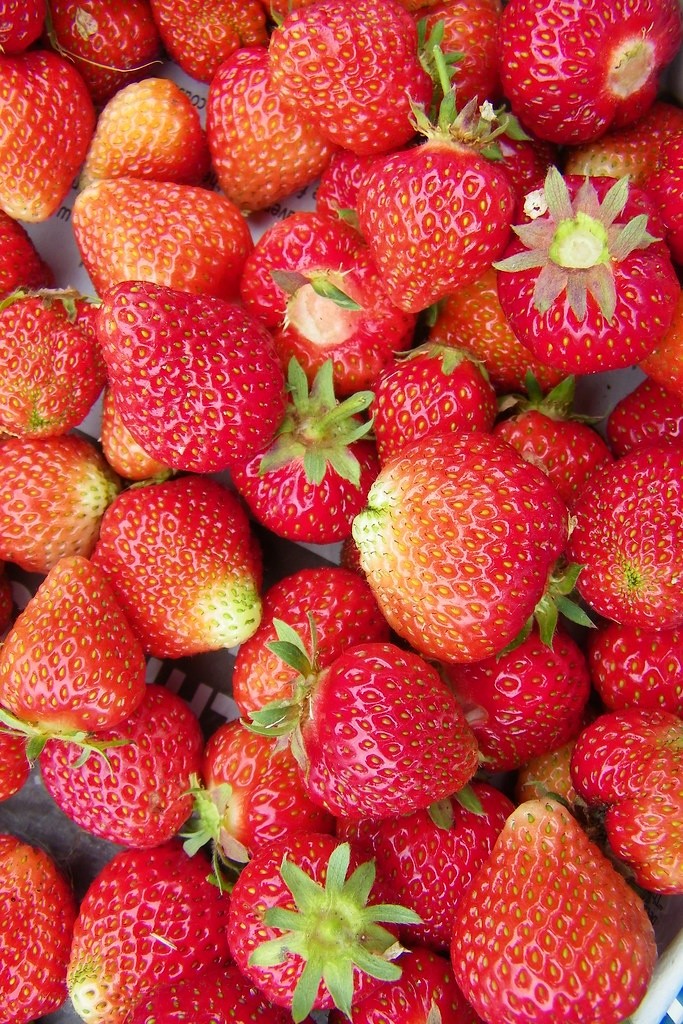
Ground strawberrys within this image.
[0,0,683,1024]
